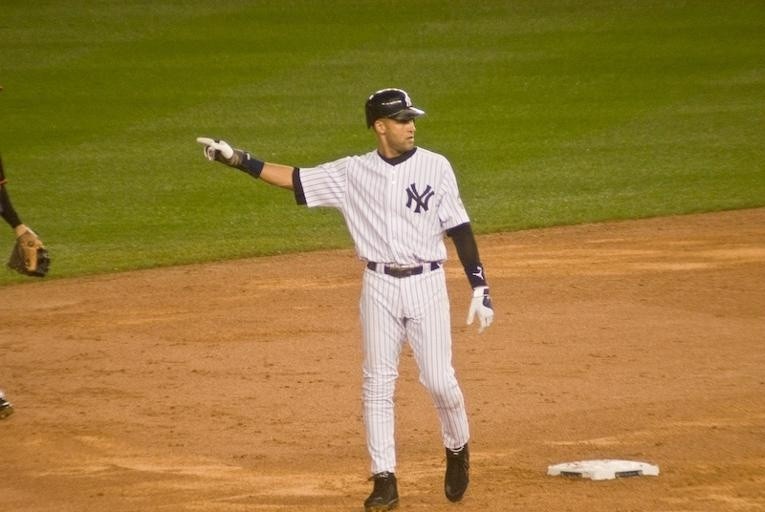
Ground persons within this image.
[196,89,494,511]
[1,164,51,417]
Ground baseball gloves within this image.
[9,232,49,277]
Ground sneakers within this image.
[364,471,399,512]
[444,442,469,503]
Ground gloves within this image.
[466,286,495,334]
[196,136,244,170]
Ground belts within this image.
[366,260,440,278]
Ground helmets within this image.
[365,88,427,128]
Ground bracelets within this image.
[463,263,487,287]
[247,156,263,179]
